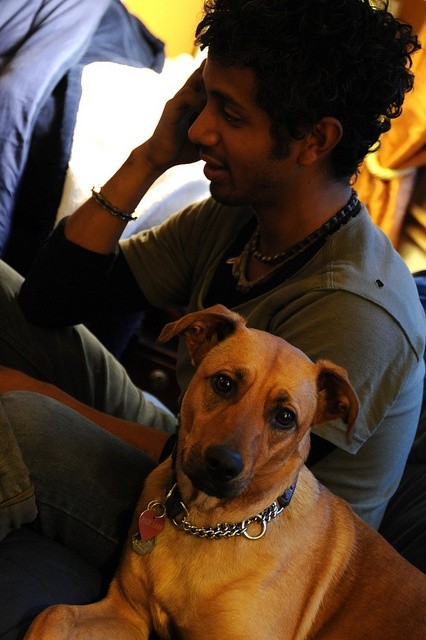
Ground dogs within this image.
[24,303,426,640]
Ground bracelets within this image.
[89,188,137,222]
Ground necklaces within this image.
[250,189,358,264]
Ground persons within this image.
[0,2,426,583]
